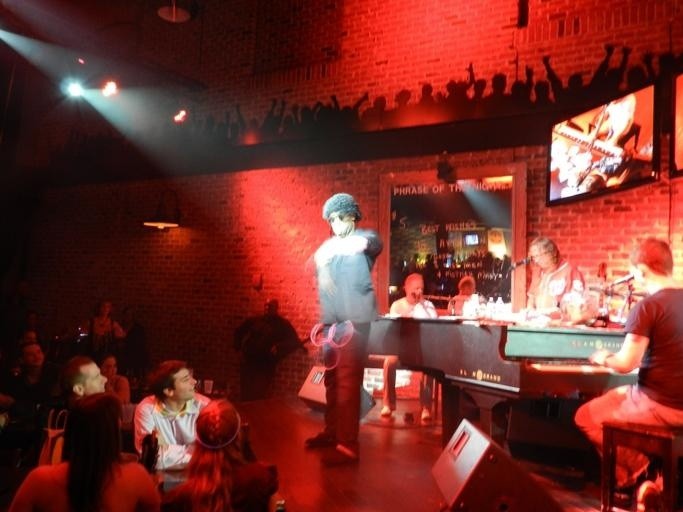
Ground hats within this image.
[195,399,242,452]
[322,192,362,221]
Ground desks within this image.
[0,349,283,511]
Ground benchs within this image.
[363,354,440,420]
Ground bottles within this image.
[455,254,461,268]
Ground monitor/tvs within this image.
[668,71,683,179]
[544,80,659,208]
[463,232,481,246]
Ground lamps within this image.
[143,187,182,231]
[434,149,459,186]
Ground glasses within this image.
[327,214,346,222]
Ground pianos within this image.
[550,125,626,163]
[367,317,639,440]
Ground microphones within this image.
[513,258,530,267]
[614,273,634,285]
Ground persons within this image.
[307,193,382,466]
[380,272,438,420]
[526,237,586,309]
[1,297,297,511]
[550,93,636,199]
[575,236,683,511]
[446,278,487,315]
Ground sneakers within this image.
[321,446,360,466]
[421,407,432,420]
[381,404,391,416]
[635,467,664,512]
[305,432,338,450]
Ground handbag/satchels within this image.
[37,408,69,467]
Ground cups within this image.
[203,380,213,394]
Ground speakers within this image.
[299,366,376,422]
[429,419,561,512]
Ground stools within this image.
[595,419,682,511]
